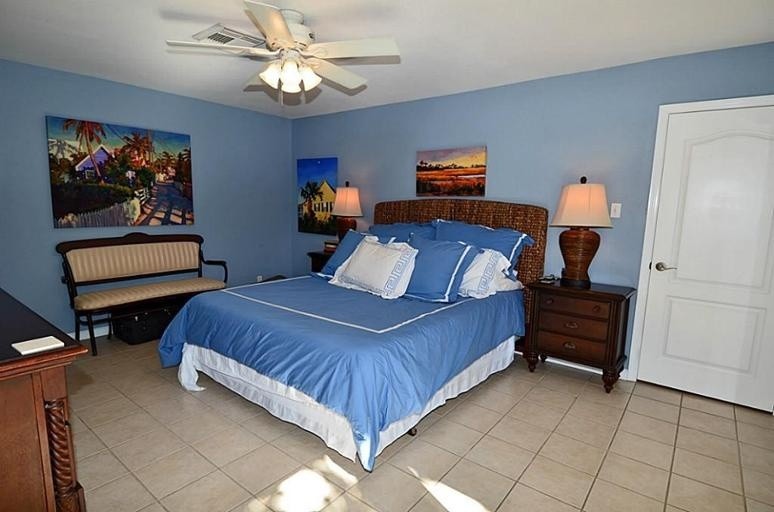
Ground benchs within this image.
[53,230,230,357]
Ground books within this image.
[323,239,338,256]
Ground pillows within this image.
[311,215,538,306]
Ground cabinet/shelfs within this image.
[0,284,94,512]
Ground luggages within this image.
[113,300,183,344]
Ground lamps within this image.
[539,174,615,292]
[324,180,364,245]
[254,51,323,98]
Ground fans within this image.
[160,1,402,101]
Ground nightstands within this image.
[304,247,336,276]
[519,271,637,394]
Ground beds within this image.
[147,195,550,480]
